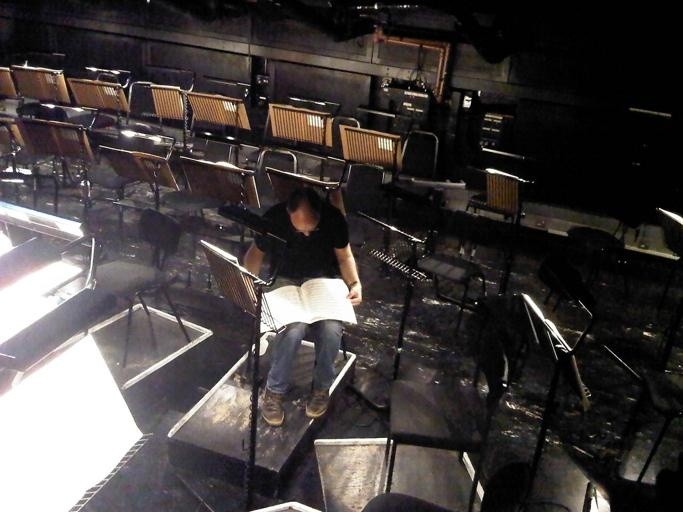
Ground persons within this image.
[242,185,363,427]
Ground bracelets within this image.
[348,280,361,289]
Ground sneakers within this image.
[260,385,285,426]
[305,377,334,418]
